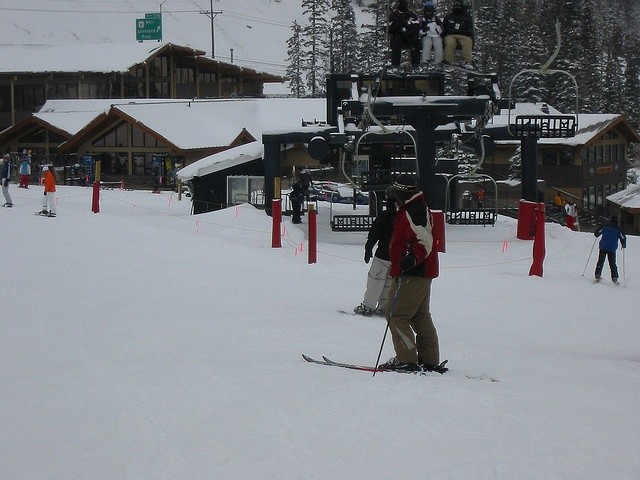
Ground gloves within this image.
[399,253,416,272]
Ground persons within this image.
[0,153,14,208]
[152,164,162,193]
[594,215,626,281]
[298,168,314,201]
[19,159,32,189]
[442,0,474,70]
[353,187,401,315]
[420,0,447,67]
[564,198,577,231]
[378,173,439,371]
[387,0,420,74]
[289,183,304,224]
[39,167,57,216]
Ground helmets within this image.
[393,175,418,193]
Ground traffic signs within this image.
[146,13,161,19]
[136,19,162,40]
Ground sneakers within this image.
[353,303,375,316]
[378,357,421,371]
[419,363,440,372]
[374,307,386,316]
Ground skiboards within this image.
[302,354,500,382]
[589,277,627,289]
